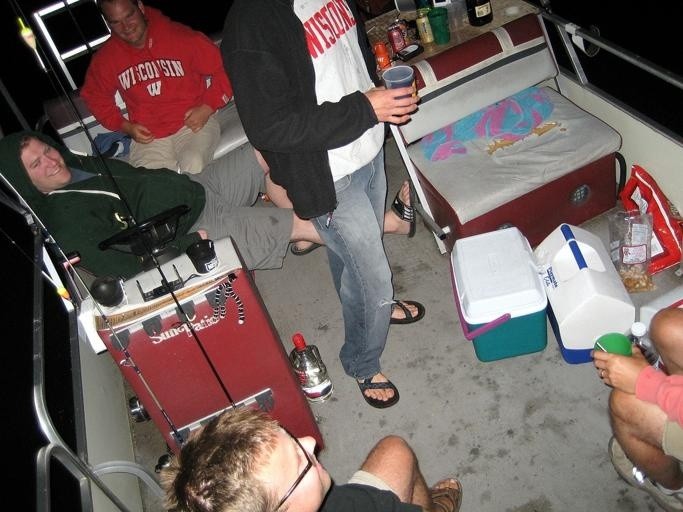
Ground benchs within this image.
[386,14,621,253]
[24,99,252,316]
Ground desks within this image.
[359,0,545,79]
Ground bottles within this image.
[629,321,659,372]
[465,0,494,26]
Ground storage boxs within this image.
[448,225,548,360]
[538,223,632,365]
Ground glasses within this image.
[271,424,316,512]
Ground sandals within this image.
[430,477,463,512]
[291,243,322,256]
[390,179,417,238]
[390,300,426,325]
[356,377,399,409]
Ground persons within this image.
[158,406,462,511]
[220,1,428,410]
[79,1,231,173]
[0,128,416,281]
[590,305,683,512]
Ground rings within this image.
[600,370,606,379]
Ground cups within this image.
[382,65,417,115]
[426,7,451,45]
[184,239,218,274]
[594,332,632,356]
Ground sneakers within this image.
[608,435,683,512]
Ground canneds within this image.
[387,15,434,54]
